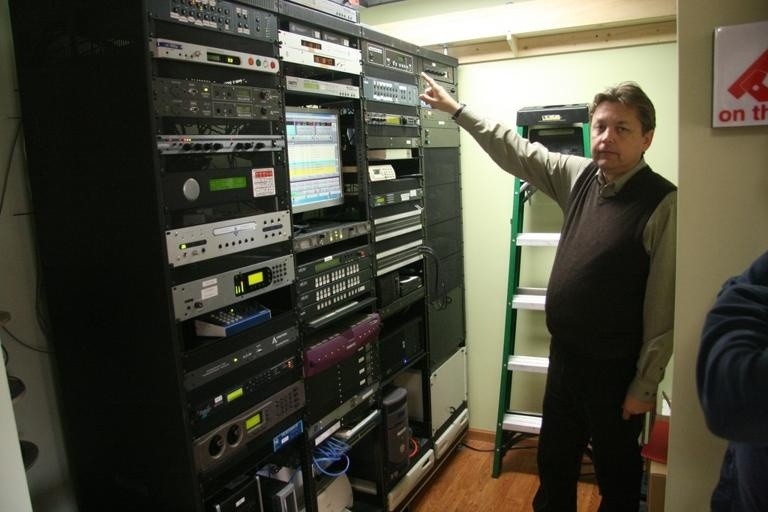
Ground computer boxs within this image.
[376,384,409,474]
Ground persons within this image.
[418,71,677,512]
[695,251,768,512]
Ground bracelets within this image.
[452,104,467,120]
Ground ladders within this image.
[491,103,593,479]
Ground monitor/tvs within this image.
[285,106,344,229]
